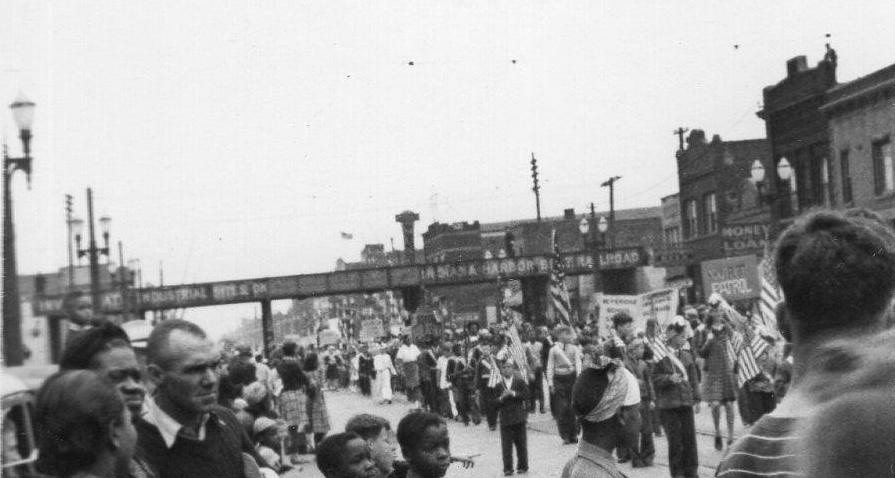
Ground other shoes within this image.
[473,420,480,424]
[517,469,526,473]
[504,470,513,475]
[714,436,722,449]
[489,426,495,430]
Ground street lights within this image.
[751,156,792,247]
[0,90,36,366]
[106,258,136,323]
[67,215,111,316]
[579,215,608,294]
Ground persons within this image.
[34,290,736,478]
[504,226,515,259]
[715,205,895,478]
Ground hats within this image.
[243,381,267,405]
[252,416,276,435]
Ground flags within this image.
[488,360,502,388]
[341,232,353,239]
[652,242,789,389]
[496,275,530,384]
[548,229,572,328]
